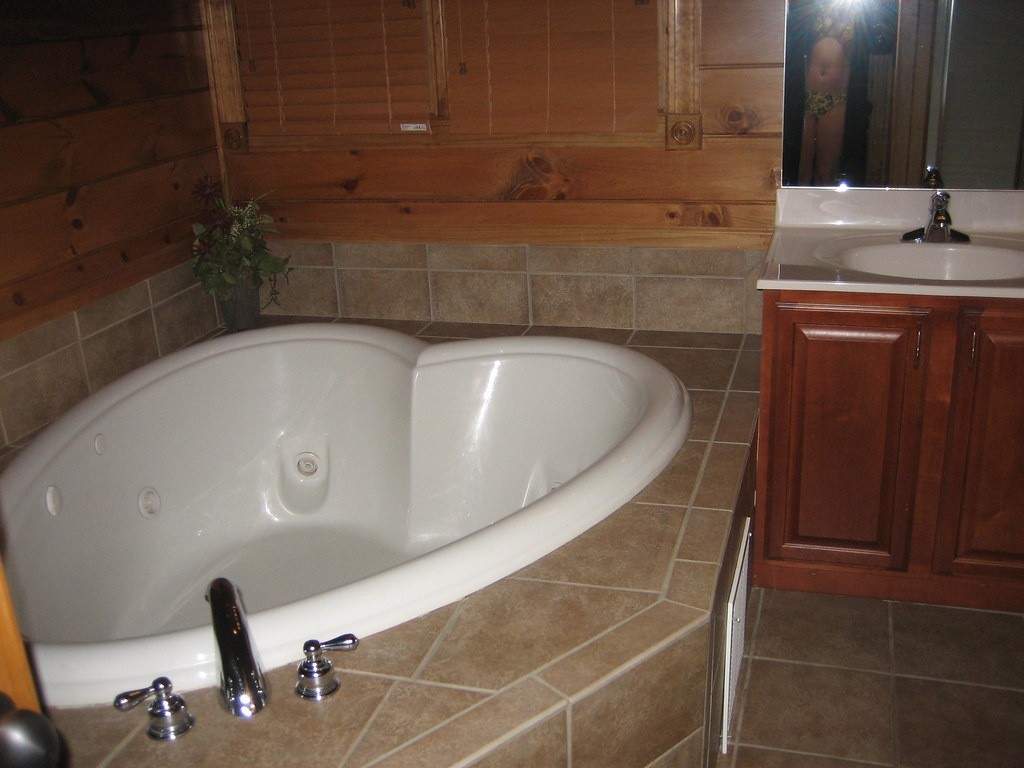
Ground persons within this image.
[795,0,861,186]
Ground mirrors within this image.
[781,0,1024,192]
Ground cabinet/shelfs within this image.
[750,289,1024,614]
[205,0,789,249]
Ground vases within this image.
[218,284,263,333]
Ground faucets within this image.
[204,577,272,718]
[923,191,952,242]
[921,168,944,189]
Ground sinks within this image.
[814,232,1024,280]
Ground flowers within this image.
[188,175,295,310]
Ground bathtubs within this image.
[0,315,765,767]
